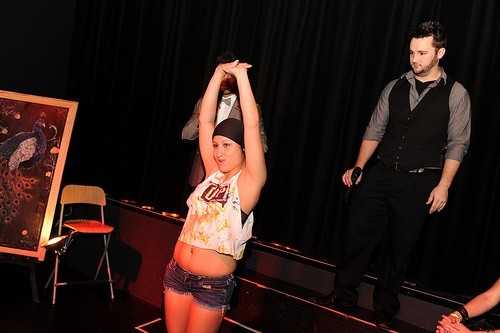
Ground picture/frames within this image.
[0,90,79,261]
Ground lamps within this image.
[40,235,70,256]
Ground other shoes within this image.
[311,290,356,308]
[372,309,394,328]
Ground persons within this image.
[181,56,268,187]
[163,59,267,333]
[311,22,470,326]
[434,277,500,333]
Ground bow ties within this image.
[221,98,231,107]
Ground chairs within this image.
[45,185,115,304]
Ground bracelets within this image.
[491,329,495,333]
[457,306,469,322]
[486,329,489,333]
[450,312,460,319]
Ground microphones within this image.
[344,167,362,201]
